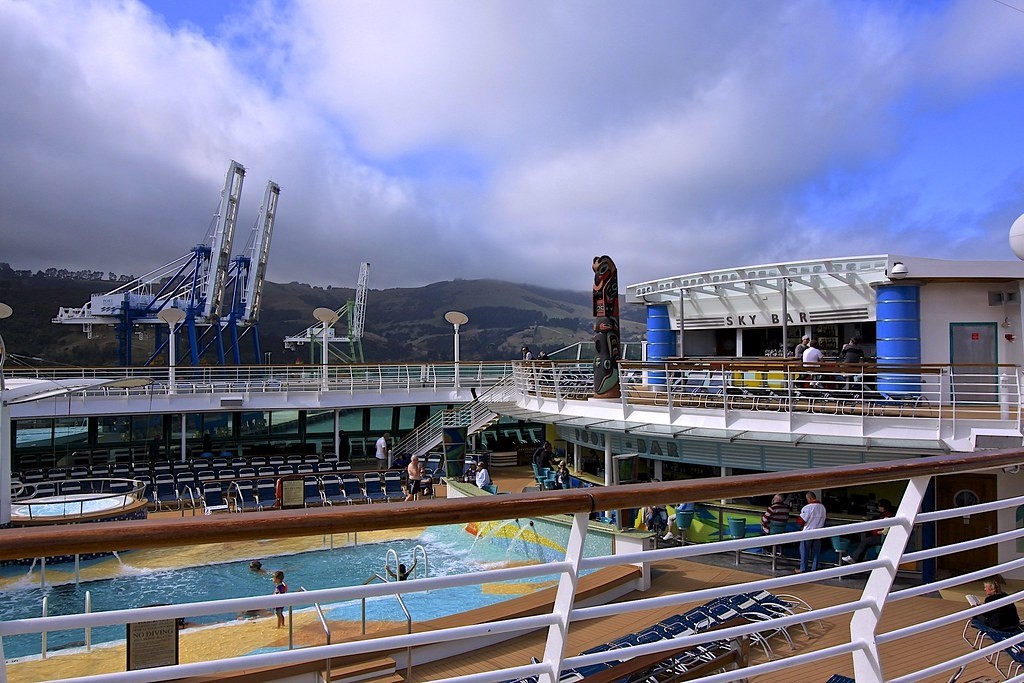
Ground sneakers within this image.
[663,532,674,540]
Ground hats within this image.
[802,334,810,341]
[850,337,858,343]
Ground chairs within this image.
[65,381,281,396]
[539,367,932,418]
[11,440,406,519]
[963,618,1024,679]
[771,520,787,571]
[415,429,589,495]
[498,587,821,683]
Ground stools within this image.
[726,516,746,564]
[832,537,849,566]
[675,511,696,545]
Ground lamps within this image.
[891,262,908,280]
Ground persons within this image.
[533,441,556,471]
[178,618,197,630]
[554,461,569,490]
[149,435,161,462]
[537,351,550,379]
[760,494,789,552]
[249,561,262,572]
[386,558,418,581]
[663,503,694,540]
[646,479,668,539]
[405,454,430,501]
[794,335,810,380]
[202,434,212,453]
[794,492,826,573]
[803,340,826,388]
[468,460,490,489]
[972,577,1020,631]
[272,571,284,629]
[836,336,860,399]
[375,433,389,469]
[339,430,349,461]
[522,346,535,363]
[842,499,896,564]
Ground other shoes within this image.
[795,568,800,573]
[842,556,855,564]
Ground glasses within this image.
[522,349,525,351]
[249,566,255,568]
